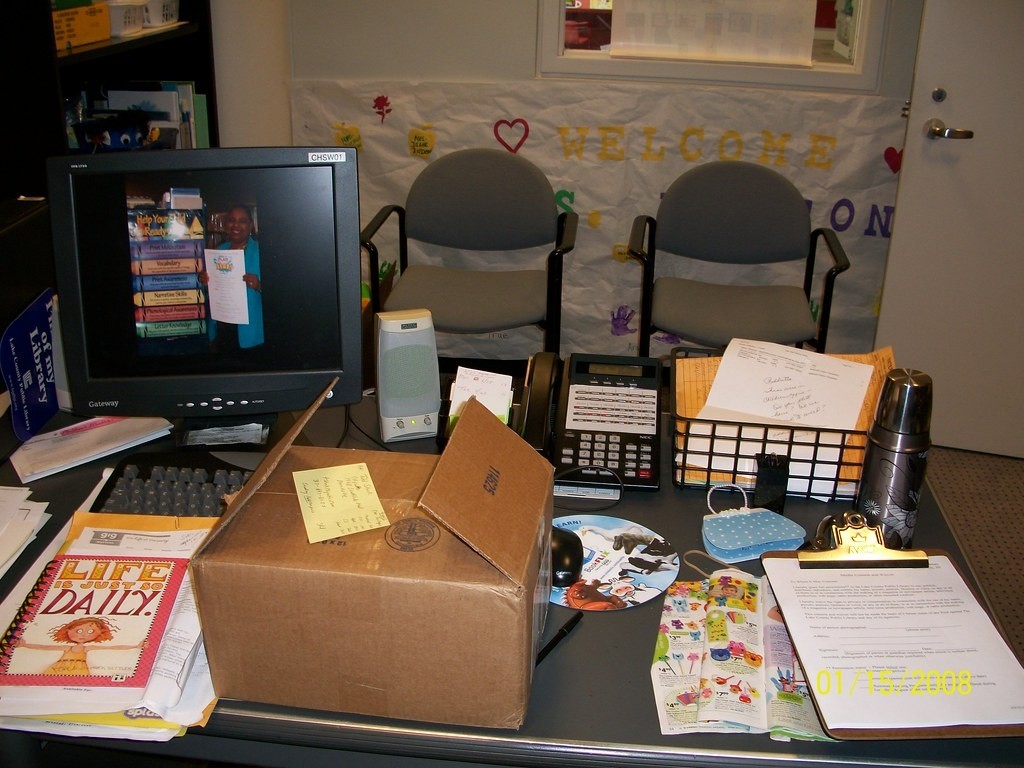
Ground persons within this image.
[197,202,265,374]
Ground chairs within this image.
[626,160,851,361]
[357,147,578,355]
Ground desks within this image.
[183,471,1024,768]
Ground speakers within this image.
[375,307,440,444]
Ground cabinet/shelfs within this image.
[0,0,220,190]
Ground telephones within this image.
[517,351,662,491]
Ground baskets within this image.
[671,346,868,503]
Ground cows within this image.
[619,536,679,576]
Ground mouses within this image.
[552,526,584,588]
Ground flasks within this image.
[855,368,933,550]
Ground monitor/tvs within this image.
[48,147,362,454]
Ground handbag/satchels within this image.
[702,483,806,564]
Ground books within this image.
[65,80,211,155]
[0,553,189,687]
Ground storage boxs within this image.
[185,376,558,731]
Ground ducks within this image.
[580,523,643,543]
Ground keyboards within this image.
[90,450,258,518]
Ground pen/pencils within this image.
[534,611,584,669]
